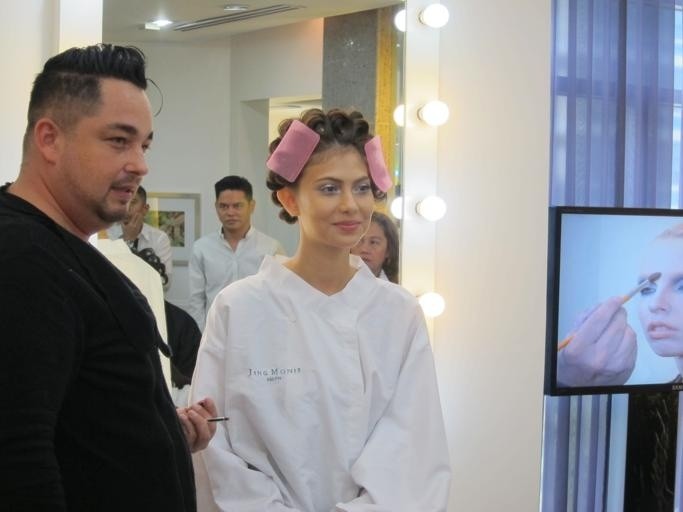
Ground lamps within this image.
[418,3,446,321]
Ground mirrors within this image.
[54,0,449,384]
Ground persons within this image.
[131,237,203,384]
[554,297,637,388]
[188,175,286,337]
[189,105,449,512]
[350,209,399,283]
[104,186,174,293]
[0,41,218,511]
[639,223,682,384]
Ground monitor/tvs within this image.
[544,206,683,396]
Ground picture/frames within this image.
[95,193,202,267]
